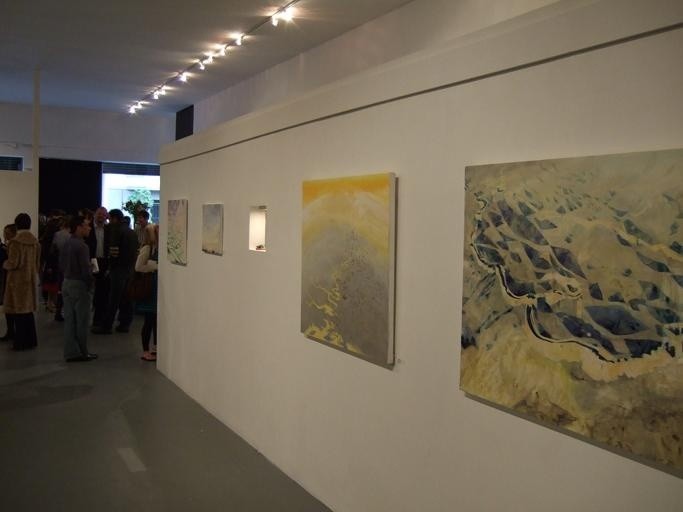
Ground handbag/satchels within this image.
[125,272,152,300]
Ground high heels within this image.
[45,305,53,313]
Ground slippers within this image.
[141,352,157,361]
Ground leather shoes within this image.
[66,353,96,361]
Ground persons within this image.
[0,203,158,364]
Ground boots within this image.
[55,293,64,321]
[0,328,15,343]
